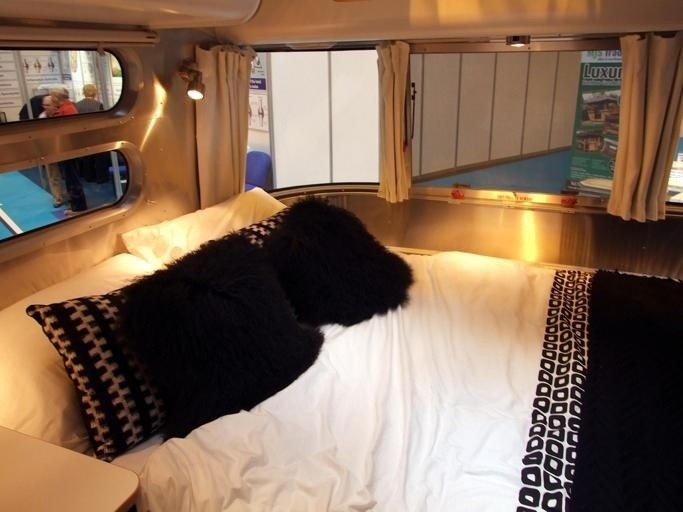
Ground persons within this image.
[48,87,87,215]
[38,96,71,207]
[74,84,108,184]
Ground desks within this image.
[1,422,146,510]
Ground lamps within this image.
[504,33,534,50]
[176,56,207,101]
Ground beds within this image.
[0,187,682,512]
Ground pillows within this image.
[232,202,300,249]
[22,276,172,463]
[116,235,322,434]
[0,247,167,462]
[260,197,414,328]
[117,186,287,265]
